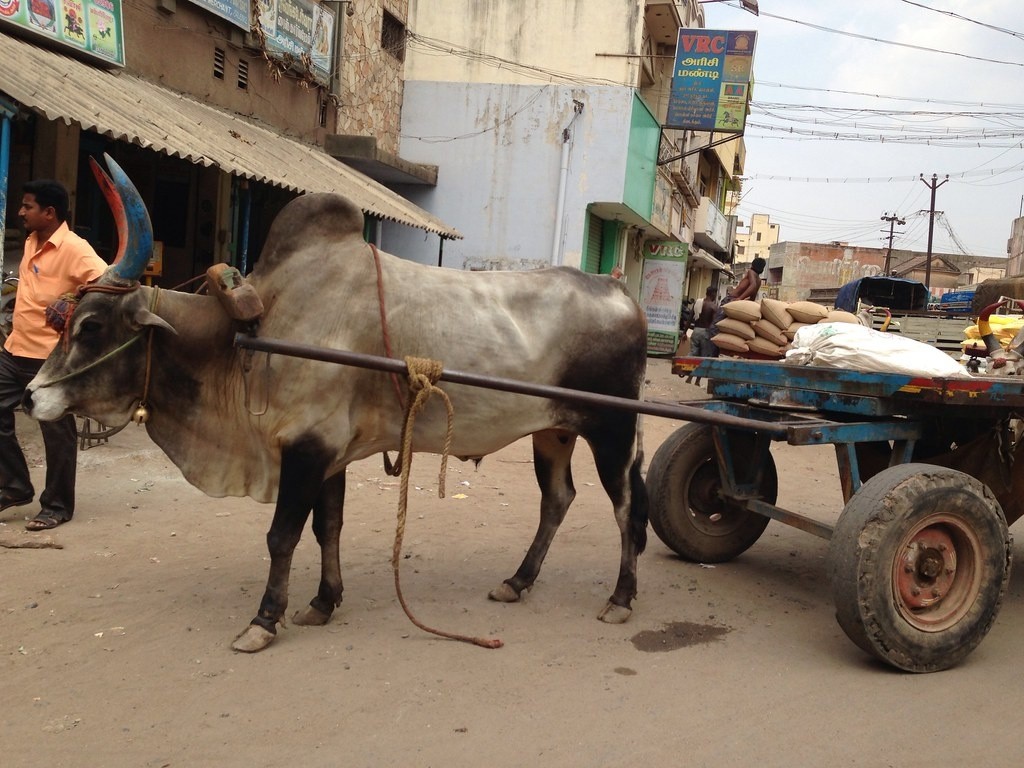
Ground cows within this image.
[32,145,656,649]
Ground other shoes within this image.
[695,379,700,386]
[686,379,691,384]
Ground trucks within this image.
[834,275,930,332]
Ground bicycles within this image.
[0,269,132,451]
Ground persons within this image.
[611,268,624,279]
[0,179,109,531]
[685,286,719,386]
[709,257,766,359]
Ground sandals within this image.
[0,491,33,512]
[25,508,64,530]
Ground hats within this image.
[752,258,765,272]
